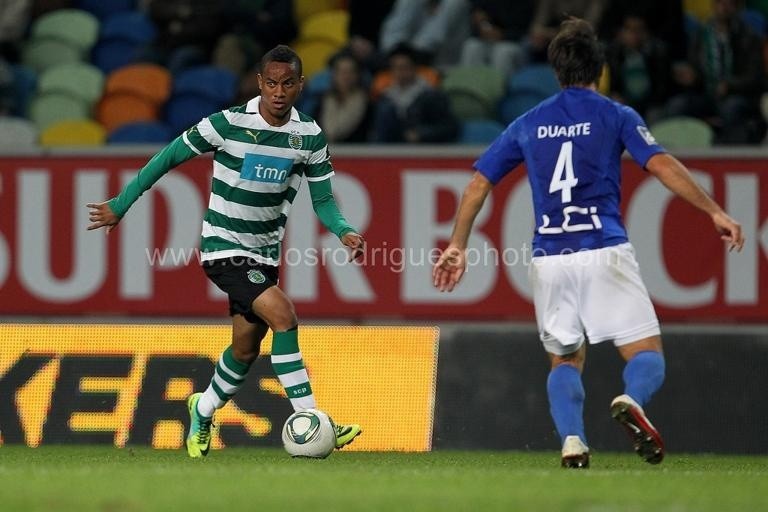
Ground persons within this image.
[431,11,743,468]
[138,0,254,65]
[315,1,765,151]
[84,43,366,458]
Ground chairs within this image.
[0,0,717,148]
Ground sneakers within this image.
[608,393,665,465]
[334,423,363,450]
[559,434,590,469]
[185,391,217,459]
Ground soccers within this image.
[281,408,337,459]
